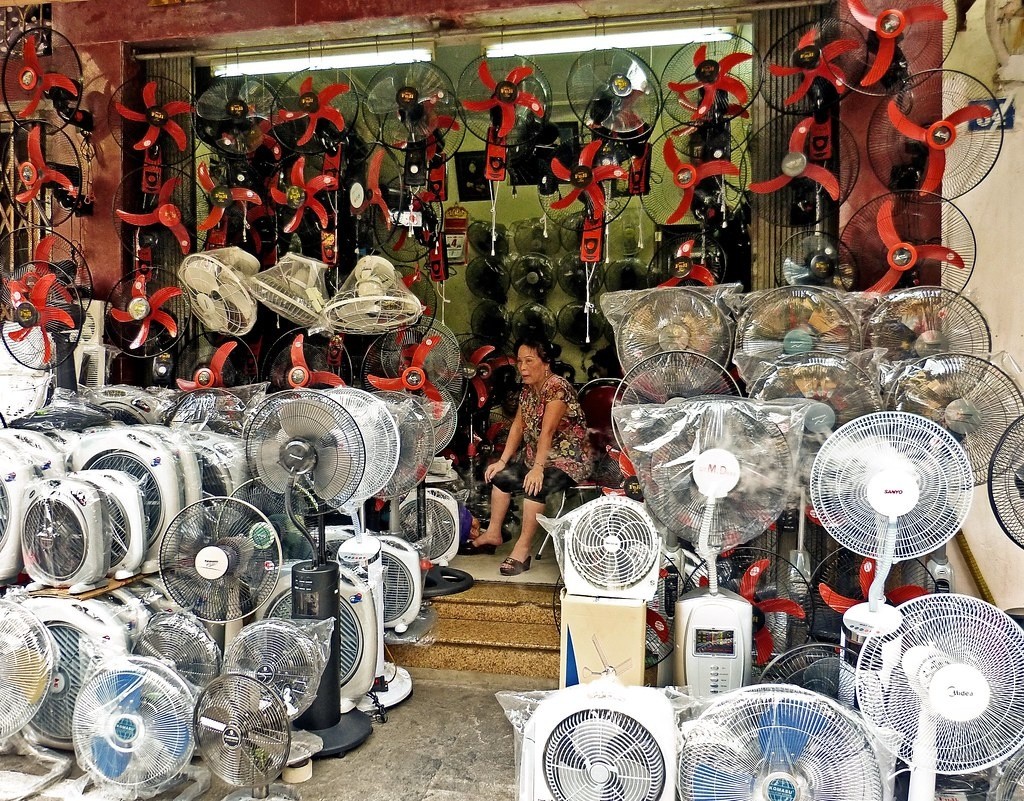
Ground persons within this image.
[457,338,594,576]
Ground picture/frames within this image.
[609,143,652,199]
[452,150,495,203]
[506,122,581,186]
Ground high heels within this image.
[456,541,497,555]
[500,555,532,576]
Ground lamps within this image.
[480,18,737,58]
[209,41,436,78]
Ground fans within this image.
[0,0,1024,800]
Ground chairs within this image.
[535,378,641,563]
